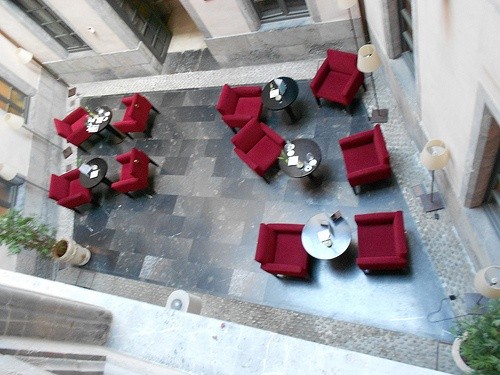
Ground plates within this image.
[283,142,317,172]
[86,107,111,131]
[269,88,282,102]
[90,164,99,178]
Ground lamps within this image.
[419,139,453,213]
[337,0,361,52]
[3,112,72,159]
[15,47,76,97]
[0,160,49,201]
[464,264,500,314]
[355,42,389,124]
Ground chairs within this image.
[337,124,393,197]
[110,92,161,144]
[110,146,160,200]
[352,209,410,272]
[307,46,368,116]
[214,83,263,134]
[44,166,100,212]
[231,117,286,185]
[254,221,309,279]
[47,108,95,153]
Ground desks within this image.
[86,102,127,145]
[299,212,353,264]
[260,76,303,129]
[274,141,326,192]
[77,158,120,198]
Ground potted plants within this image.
[0,206,92,268]
[447,299,500,375]
